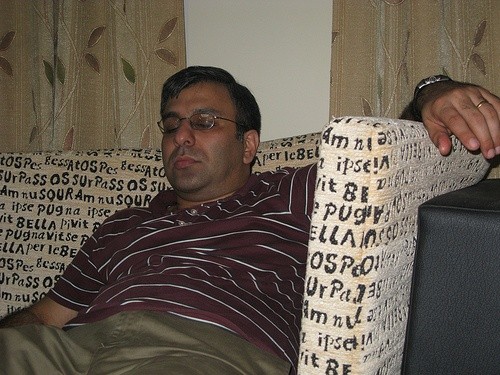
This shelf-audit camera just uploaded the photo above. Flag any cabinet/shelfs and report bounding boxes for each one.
[402,178,500,375]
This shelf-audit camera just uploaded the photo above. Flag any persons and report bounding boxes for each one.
[0,66,500,375]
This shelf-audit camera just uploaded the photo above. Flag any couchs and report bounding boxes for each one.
[0,116,495,375]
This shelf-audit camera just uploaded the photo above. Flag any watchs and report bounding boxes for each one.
[412,74,453,122]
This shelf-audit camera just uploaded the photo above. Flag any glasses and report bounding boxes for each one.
[157,113,237,134]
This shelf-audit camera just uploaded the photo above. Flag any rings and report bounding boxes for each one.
[476,99,489,109]
